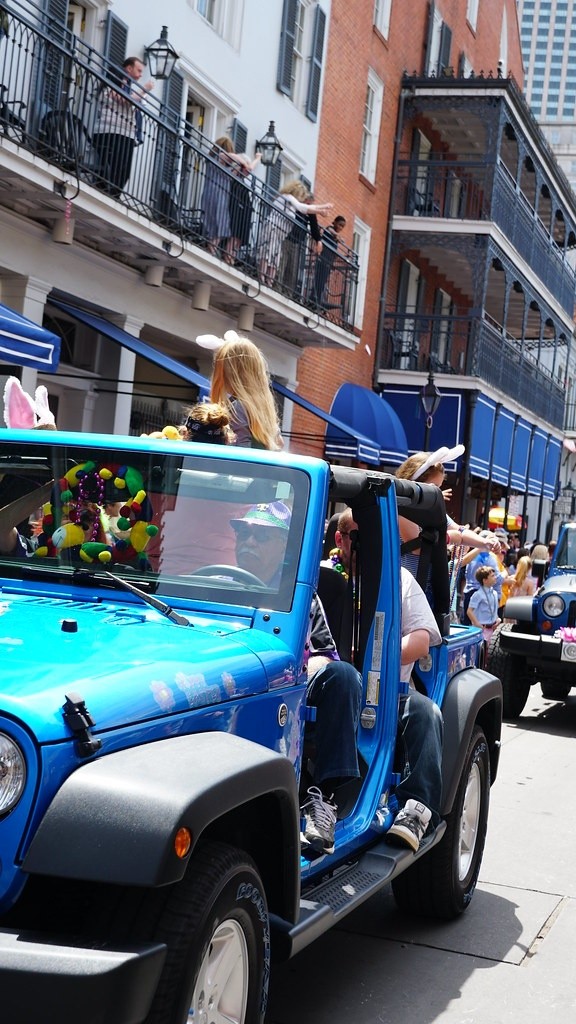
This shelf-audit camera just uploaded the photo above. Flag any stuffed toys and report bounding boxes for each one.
[33,460,159,571]
[52,522,93,563]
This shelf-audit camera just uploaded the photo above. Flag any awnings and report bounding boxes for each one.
[47,296,211,403]
[268,378,380,466]
[325,383,409,466]
[0,303,61,374]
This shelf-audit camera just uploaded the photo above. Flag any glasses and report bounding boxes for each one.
[235,528,270,542]
[340,530,358,539]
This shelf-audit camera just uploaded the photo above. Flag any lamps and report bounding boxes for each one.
[255,120,283,167]
[562,482,576,497]
[50,217,76,246]
[142,25,181,81]
[144,265,165,287]
[191,283,212,312]
[236,305,256,334]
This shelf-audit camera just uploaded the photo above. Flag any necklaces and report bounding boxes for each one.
[76,472,105,542]
[330,548,361,615]
[447,526,464,612]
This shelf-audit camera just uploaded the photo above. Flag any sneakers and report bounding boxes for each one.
[300,786,338,854]
[387,799,432,852]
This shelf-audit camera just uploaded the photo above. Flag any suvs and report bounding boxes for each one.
[485,523,576,719]
[0,426,507,1024]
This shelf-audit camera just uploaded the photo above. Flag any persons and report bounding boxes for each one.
[320,507,444,852]
[92,57,155,199]
[0,329,284,580]
[207,501,362,862]
[200,136,262,266]
[258,180,347,316]
[462,526,558,665]
[394,444,502,613]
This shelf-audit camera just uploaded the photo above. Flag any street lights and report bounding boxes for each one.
[419,370,443,451]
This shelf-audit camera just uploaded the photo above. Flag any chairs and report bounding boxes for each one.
[384,327,419,372]
[427,353,456,374]
[154,181,206,236]
[316,566,353,664]
[38,110,97,175]
[406,181,440,217]
[0,83,28,144]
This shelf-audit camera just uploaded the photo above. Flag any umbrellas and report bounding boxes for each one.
[482,503,527,530]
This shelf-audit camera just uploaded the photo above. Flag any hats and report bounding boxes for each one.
[531,545,549,561]
[496,531,510,549]
[494,528,509,535]
[230,501,292,533]
